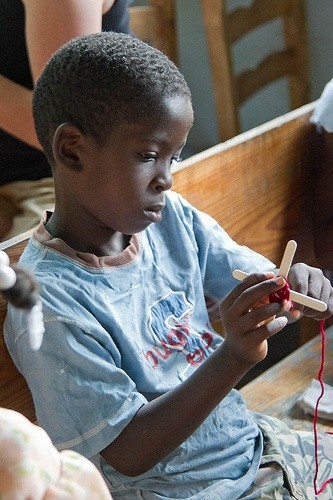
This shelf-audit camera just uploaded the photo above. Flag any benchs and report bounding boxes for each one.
[0,98,333,437]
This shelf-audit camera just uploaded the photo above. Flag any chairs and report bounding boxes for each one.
[129,0,180,74]
[201,0,310,144]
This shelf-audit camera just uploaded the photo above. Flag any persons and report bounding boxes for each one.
[0,0,132,243]
[308,73,333,273]
[3,29,333,500]
